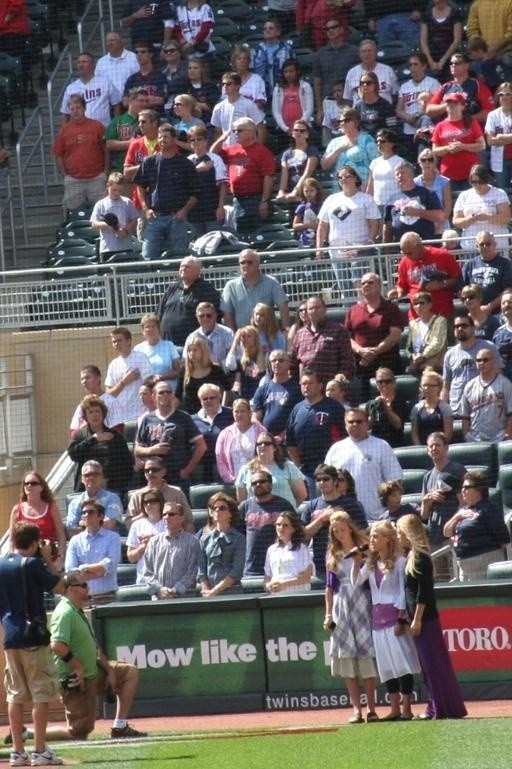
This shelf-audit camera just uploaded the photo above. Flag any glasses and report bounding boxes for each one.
[24,481,40,486]
[81,509,97,515]
[336,174,354,180]
[199,313,215,319]
[361,81,372,85]
[341,118,352,122]
[239,260,256,265]
[294,128,306,133]
[272,359,287,363]
[144,468,163,473]
[164,48,177,54]
[377,379,392,385]
[377,140,390,146]
[257,441,273,446]
[316,477,333,481]
[498,91,509,97]
[203,397,217,400]
[158,390,171,394]
[252,479,271,486]
[450,62,463,66]
[476,358,488,363]
[455,324,470,328]
[478,242,489,247]
[462,296,475,301]
[163,513,181,517]
[232,129,248,133]
[71,583,88,589]
[420,158,435,163]
[213,506,228,511]
[142,498,159,505]
[362,281,376,285]
[222,83,234,86]
[325,25,340,31]
[414,302,427,306]
[298,309,306,312]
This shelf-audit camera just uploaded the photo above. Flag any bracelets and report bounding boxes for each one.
[325,613,334,618]
[396,617,409,625]
[59,650,73,663]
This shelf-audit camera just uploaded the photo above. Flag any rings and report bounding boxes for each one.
[323,624,326,628]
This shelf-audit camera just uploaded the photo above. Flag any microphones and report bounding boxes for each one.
[344,544,368,559]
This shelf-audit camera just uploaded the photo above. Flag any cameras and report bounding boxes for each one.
[38,541,59,558]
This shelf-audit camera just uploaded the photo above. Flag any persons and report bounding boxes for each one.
[390,512,470,719]
[2,573,149,744]
[343,517,424,720]
[46,0,511,606]
[0,523,66,767]
[6,468,66,573]
[1,1,32,60]
[321,511,383,723]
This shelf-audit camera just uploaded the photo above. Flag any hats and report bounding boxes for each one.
[445,93,465,105]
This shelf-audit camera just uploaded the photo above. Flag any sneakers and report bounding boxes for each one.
[349,714,364,723]
[366,712,379,721]
[380,713,399,721]
[4,725,26,743]
[28,752,63,766]
[111,724,148,739]
[10,751,30,766]
[401,714,414,720]
[418,713,432,719]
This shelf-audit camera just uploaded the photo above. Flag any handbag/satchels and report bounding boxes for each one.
[23,619,52,646]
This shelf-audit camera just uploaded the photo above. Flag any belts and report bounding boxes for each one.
[326,97,334,100]
